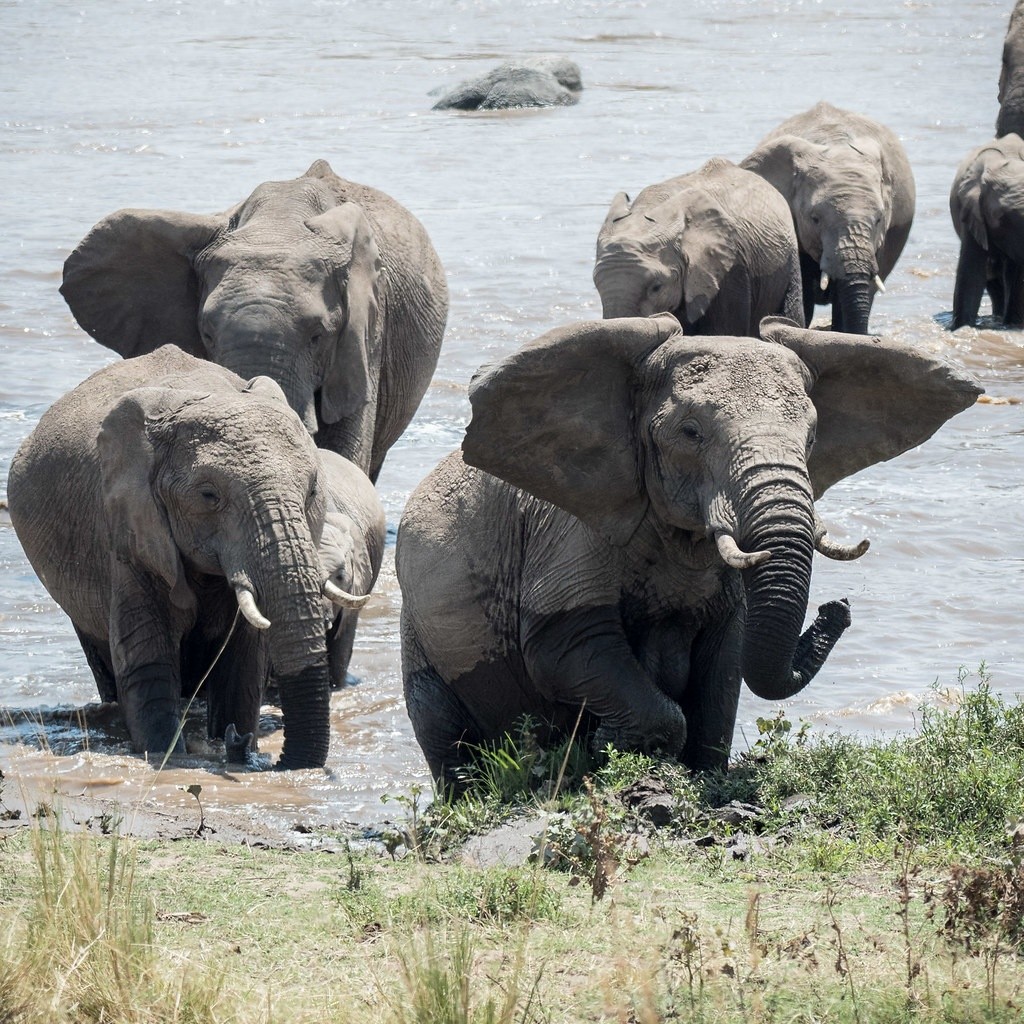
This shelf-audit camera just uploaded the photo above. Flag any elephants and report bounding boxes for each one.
[945,0,1024,331]
[394,98,986,817]
[5,157,450,774]
